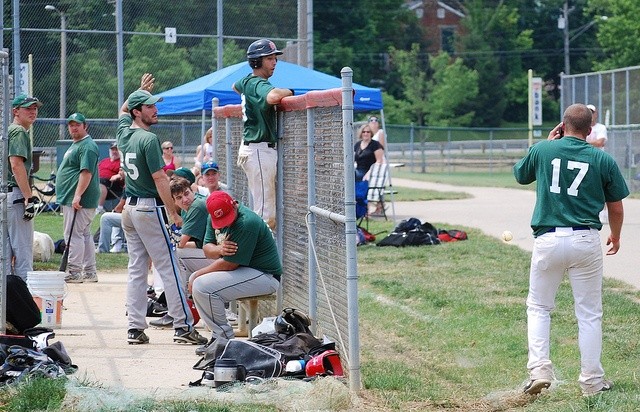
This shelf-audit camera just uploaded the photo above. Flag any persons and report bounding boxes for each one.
[165,167,206,220]
[96,167,125,253]
[96,143,121,214]
[200,161,229,197]
[115,72,208,344]
[187,191,283,356]
[368,116,389,219]
[587,103,610,227]
[161,141,181,173]
[513,102,630,397]
[231,39,295,230]
[6,93,43,282]
[353,124,385,182]
[193,126,212,167]
[54,113,101,283]
[149,177,217,327]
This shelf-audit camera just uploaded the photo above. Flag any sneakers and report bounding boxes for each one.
[65,273,83,283]
[128,329,149,344]
[523,380,551,395]
[82,272,98,282]
[174,328,208,345]
[598,380,615,391]
[109,243,125,253]
[195,336,216,357]
[149,314,174,330]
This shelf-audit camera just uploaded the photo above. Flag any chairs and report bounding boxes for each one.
[357,161,389,228]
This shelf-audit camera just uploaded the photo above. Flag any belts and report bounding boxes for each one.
[548,225,590,231]
[244,141,275,147]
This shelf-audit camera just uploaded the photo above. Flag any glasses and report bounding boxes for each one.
[16,97,39,108]
[163,147,173,150]
[370,119,378,121]
[362,131,370,133]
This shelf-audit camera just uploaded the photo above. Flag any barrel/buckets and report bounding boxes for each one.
[26,271,67,328]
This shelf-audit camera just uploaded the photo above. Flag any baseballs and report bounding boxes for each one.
[502,230,513,241]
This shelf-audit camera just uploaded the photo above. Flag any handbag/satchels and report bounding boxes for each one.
[376,232,408,246]
[396,218,439,245]
[221,332,322,379]
[437,230,467,242]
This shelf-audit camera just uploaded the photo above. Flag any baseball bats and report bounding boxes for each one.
[59,207,78,272]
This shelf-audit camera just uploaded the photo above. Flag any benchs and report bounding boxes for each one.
[227,297,276,338]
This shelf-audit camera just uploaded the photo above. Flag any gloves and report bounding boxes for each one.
[169,224,182,251]
[23,198,34,221]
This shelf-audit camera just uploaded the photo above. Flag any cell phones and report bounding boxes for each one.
[555,124,563,140]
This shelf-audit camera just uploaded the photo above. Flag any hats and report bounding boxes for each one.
[12,95,42,108]
[167,167,195,183]
[66,112,85,123]
[128,90,164,110]
[586,104,595,112]
[110,142,117,148]
[206,190,235,229]
[201,162,219,175]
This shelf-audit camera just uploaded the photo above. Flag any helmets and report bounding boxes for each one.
[247,38,284,68]
[305,350,343,377]
